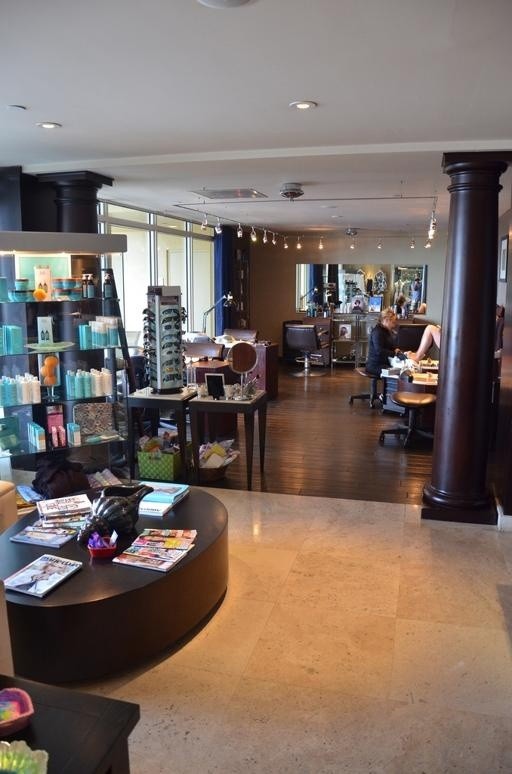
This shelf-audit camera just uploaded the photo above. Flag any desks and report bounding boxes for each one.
[0,672,140,774]
[245,340,279,401]
[0,479,227,674]
[127,386,267,483]
[193,361,230,385]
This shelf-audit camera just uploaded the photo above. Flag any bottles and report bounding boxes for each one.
[52,425,66,447]
[65,367,113,399]
[26,420,46,451]
[67,422,82,446]
[78,315,119,351]
[0,277,8,303]
[0,372,42,407]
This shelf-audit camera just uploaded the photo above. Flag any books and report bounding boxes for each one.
[111,529,198,573]
[87,469,122,490]
[134,481,190,517]
[16,486,45,516]
[10,493,93,549]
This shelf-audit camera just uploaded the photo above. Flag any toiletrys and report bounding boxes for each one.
[27,422,81,453]
[66,368,112,399]
[78,316,117,351]
[8,277,31,302]
[102,272,112,297]
[51,277,82,300]
[0,372,41,405]
[80,271,95,299]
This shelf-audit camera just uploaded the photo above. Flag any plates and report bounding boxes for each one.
[24,342,75,352]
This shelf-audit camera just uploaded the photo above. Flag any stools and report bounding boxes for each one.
[349,368,383,408]
[0,479,17,531]
[379,392,437,447]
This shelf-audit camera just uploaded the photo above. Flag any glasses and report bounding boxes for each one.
[163,367,179,372]
[163,374,182,382]
[145,367,156,374]
[143,326,154,333]
[161,316,178,323]
[145,359,157,365]
[163,325,179,330]
[143,342,150,346]
[179,312,185,316]
[161,334,179,341]
[163,350,178,355]
[183,362,186,368]
[177,330,186,335]
[162,309,179,315]
[179,317,185,324]
[143,317,155,324]
[142,349,156,358]
[179,307,185,312]
[162,342,179,349]
[183,347,187,353]
[143,334,155,340]
[142,309,155,317]
[148,375,157,382]
[162,358,179,366]
[182,356,185,360]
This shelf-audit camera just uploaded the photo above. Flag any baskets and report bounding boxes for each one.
[190,465,227,481]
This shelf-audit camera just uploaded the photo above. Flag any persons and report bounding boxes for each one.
[395,278,426,318]
[3,553,84,599]
[14,558,71,592]
[352,310,441,402]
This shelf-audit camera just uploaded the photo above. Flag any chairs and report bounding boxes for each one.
[392,318,434,350]
[224,328,258,340]
[182,344,225,359]
[283,320,328,378]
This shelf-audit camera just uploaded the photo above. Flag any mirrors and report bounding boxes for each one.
[226,342,258,400]
[295,263,427,315]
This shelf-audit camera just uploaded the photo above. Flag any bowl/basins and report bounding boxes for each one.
[87,536,118,559]
[0,687,36,737]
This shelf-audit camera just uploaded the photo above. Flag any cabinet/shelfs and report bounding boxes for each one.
[331,313,380,376]
[302,317,330,367]
[1,230,145,511]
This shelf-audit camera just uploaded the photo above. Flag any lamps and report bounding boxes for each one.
[300,287,318,308]
[280,183,304,202]
[200,210,438,250]
[203,292,237,333]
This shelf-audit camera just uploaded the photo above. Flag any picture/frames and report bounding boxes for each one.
[339,323,351,339]
[499,233,509,282]
